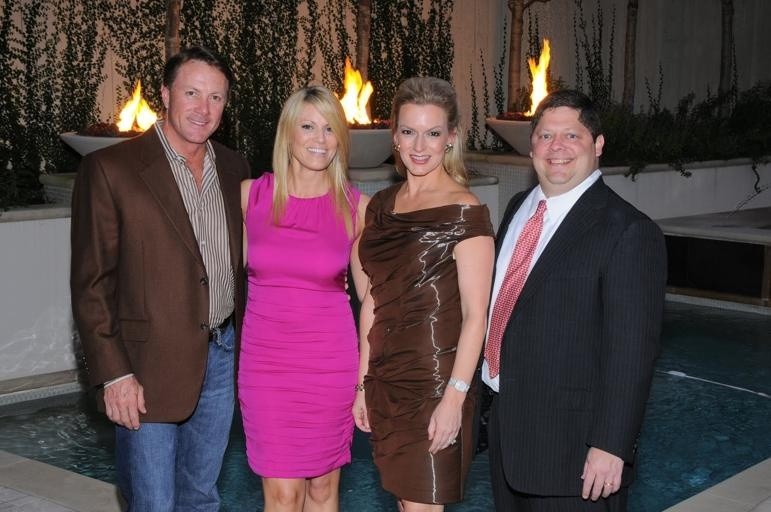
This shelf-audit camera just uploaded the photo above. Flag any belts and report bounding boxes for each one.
[210,316,232,342]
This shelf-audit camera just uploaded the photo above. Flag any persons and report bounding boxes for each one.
[348,73,497,512]
[68,46,252,512]
[237,84,374,512]
[474,89,670,510]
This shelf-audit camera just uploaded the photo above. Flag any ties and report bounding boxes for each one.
[483,200,547,379]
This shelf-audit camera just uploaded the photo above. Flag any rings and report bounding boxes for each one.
[448,437,458,445]
[604,480,616,487]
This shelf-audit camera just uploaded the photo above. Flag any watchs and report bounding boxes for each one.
[446,377,471,395]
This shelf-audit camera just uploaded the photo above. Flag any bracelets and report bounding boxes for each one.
[354,381,367,393]
